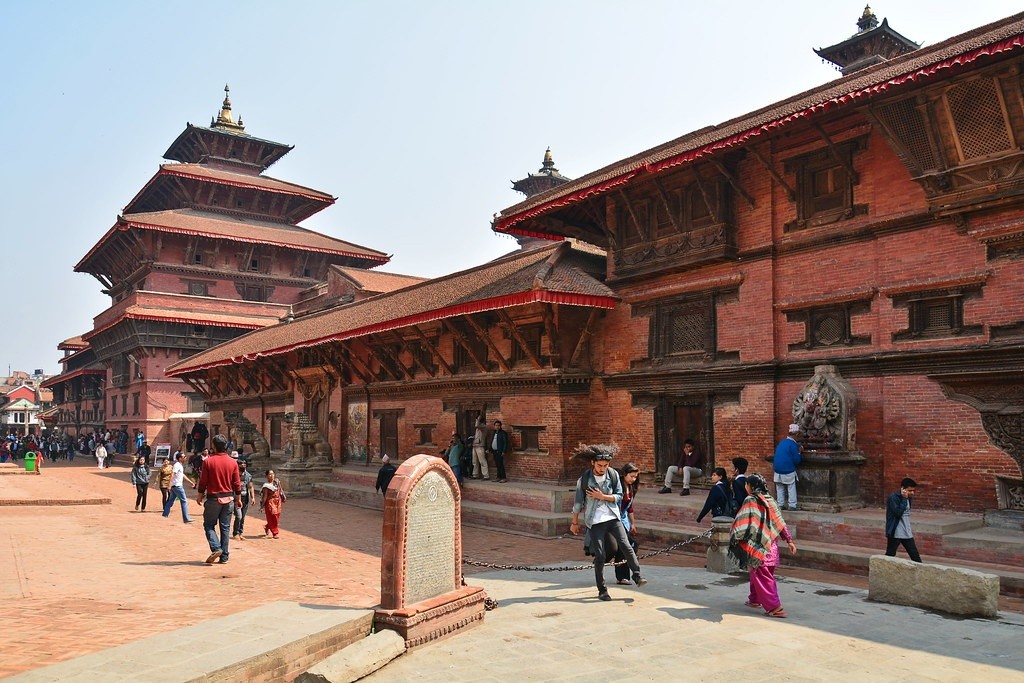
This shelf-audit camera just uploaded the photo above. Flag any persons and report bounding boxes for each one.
[696,468,734,524]
[485,421,508,483]
[467,415,490,480]
[439,434,477,488]
[195,433,244,564]
[727,472,796,617]
[658,439,703,495]
[375,452,396,498]
[884,477,922,563]
[729,458,750,573]
[568,441,648,601]
[583,462,639,585]
[774,424,804,511]
[0,420,287,539]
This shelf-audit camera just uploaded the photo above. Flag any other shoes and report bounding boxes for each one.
[218,559,228,563]
[234,534,246,540]
[263,525,269,535]
[765,609,787,618]
[273,534,279,539]
[658,487,672,494]
[448,471,512,488]
[632,574,647,586]
[744,601,762,607]
[206,550,222,562]
[680,489,690,495]
[599,592,611,601]
[135,506,146,512]
[184,518,195,523]
[779,506,801,510]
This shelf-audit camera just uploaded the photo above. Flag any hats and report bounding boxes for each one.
[788,424,799,432]
[230,451,239,458]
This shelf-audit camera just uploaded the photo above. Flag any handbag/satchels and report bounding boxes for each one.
[621,511,631,532]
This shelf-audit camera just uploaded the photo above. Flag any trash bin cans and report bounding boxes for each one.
[24,452,37,471]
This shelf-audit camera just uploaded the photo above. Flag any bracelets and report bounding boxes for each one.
[572,522,579,526]
